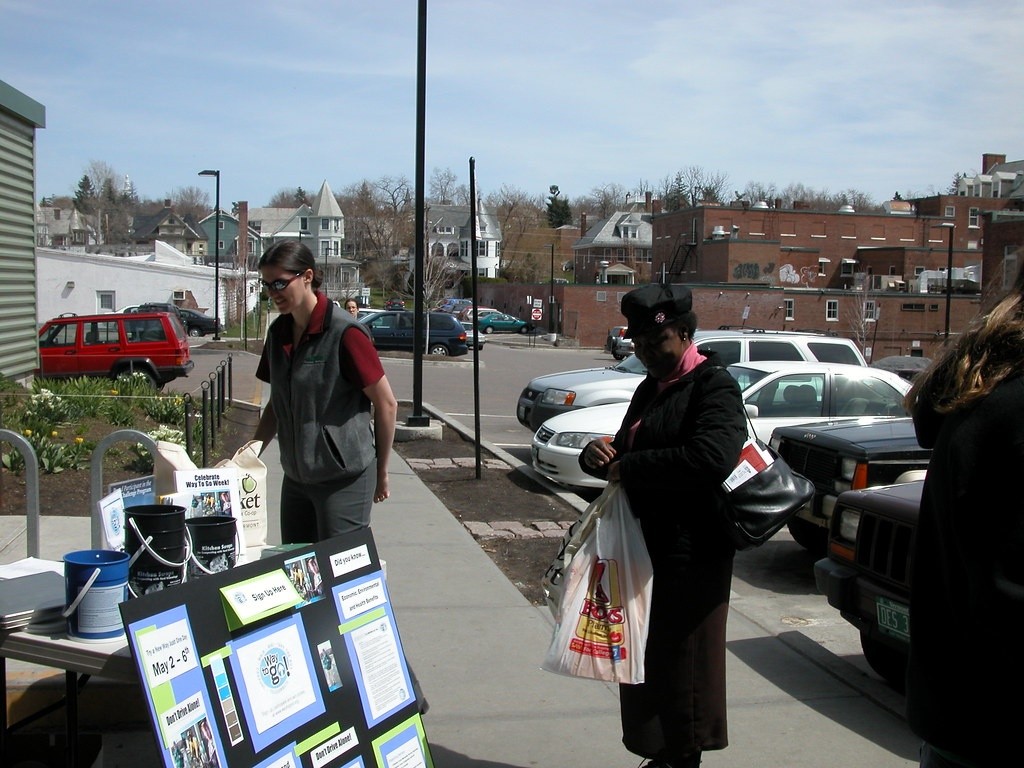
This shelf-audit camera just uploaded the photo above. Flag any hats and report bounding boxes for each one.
[621,283,692,340]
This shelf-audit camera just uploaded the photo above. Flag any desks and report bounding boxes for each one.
[0,624,163,768]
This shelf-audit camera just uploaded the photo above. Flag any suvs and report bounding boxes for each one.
[606,325,635,360]
[38,311,195,395]
[515,328,874,437]
[358,310,469,357]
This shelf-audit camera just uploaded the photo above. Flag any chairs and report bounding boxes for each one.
[775,385,798,417]
[791,385,820,416]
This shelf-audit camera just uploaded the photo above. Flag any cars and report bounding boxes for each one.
[384,298,406,310]
[868,355,932,383]
[101,302,224,343]
[356,307,384,325]
[765,416,934,558]
[430,297,535,351]
[529,359,920,495]
[810,468,931,688]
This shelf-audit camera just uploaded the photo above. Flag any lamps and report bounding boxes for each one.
[67,281,75,288]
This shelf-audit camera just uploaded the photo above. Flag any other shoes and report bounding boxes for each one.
[638,755,700,768]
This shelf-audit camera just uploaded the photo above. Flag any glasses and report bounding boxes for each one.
[261,269,307,293]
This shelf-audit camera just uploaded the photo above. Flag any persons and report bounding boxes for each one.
[251,239,397,544]
[193,493,231,517]
[344,298,359,318]
[902,257,1024,768]
[171,720,218,768]
[578,281,748,768]
[286,556,323,599]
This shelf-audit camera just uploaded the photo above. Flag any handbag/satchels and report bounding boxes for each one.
[688,365,815,547]
[541,480,655,683]
[208,439,269,546]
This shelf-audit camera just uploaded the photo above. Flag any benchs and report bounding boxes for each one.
[837,398,906,417]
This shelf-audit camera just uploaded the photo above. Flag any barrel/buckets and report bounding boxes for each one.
[123,504,194,601]
[63,548,131,644]
[183,515,240,584]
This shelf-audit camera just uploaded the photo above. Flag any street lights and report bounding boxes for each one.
[325,247,333,293]
[197,169,220,340]
[299,230,311,242]
[939,222,954,346]
